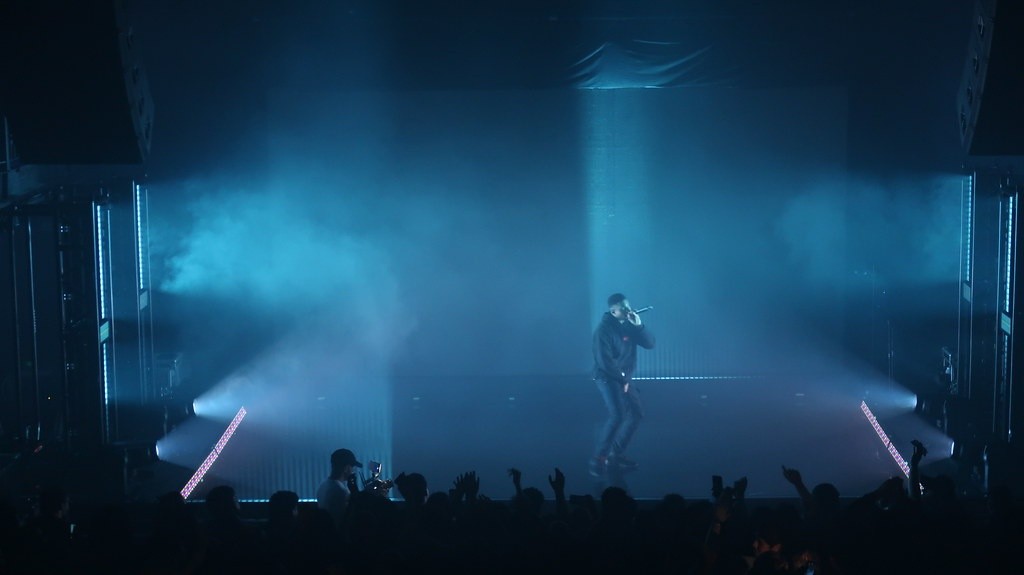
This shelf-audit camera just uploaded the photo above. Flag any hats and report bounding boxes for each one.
[333,447,363,468]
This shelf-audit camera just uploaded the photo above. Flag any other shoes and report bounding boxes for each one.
[587,457,603,476]
[605,455,639,468]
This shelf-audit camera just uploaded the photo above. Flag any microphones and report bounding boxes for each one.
[628,305,654,317]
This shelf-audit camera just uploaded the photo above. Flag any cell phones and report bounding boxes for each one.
[712,476,723,499]
[911,439,928,456]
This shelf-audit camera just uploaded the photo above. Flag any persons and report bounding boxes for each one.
[314,448,364,525]
[584,293,656,477]
[0,443,1024,575]
